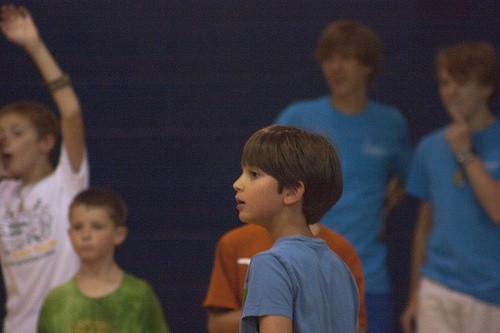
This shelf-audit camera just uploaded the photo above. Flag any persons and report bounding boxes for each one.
[270,20,417,333]
[37,188,165,332]
[205,222,366,332]
[0,3,89,333]
[402,40,500,332]
[233,125,360,333]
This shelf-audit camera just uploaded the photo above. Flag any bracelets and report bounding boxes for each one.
[455,146,477,164]
[46,74,72,93]
